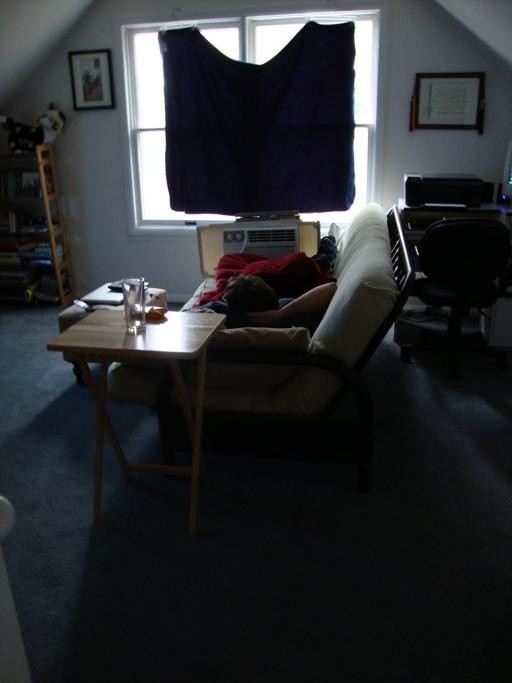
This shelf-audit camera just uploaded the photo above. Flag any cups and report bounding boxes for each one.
[122,279,147,332]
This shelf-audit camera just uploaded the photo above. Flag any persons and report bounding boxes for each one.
[177,272,338,336]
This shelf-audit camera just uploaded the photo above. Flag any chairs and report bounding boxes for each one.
[401,216,511,360]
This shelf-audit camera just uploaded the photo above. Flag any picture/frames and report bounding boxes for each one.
[69,49,116,112]
[410,70,486,135]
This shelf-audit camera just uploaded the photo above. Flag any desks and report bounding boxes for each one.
[46,307,227,541]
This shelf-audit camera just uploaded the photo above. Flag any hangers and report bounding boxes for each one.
[306,1,358,25]
[156,7,200,38]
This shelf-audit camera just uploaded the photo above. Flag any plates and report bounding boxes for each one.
[130,304,166,320]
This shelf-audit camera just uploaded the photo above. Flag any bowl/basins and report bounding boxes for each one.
[129,288,168,309]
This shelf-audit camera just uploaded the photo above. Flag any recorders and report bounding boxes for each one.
[222,221,298,257]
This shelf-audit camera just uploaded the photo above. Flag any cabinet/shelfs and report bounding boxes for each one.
[1,141,77,311]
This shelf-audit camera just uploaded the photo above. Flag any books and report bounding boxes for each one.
[1,167,72,304]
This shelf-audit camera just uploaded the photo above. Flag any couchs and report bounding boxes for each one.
[157,203,408,469]
